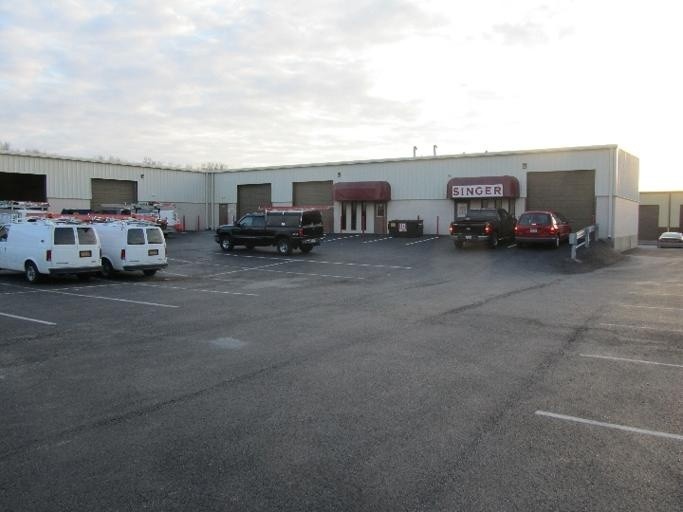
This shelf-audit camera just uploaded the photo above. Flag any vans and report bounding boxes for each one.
[0,199,184,284]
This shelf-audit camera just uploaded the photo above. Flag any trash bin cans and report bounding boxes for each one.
[388,220,423,237]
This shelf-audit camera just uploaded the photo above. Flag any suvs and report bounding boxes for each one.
[214,208,327,257]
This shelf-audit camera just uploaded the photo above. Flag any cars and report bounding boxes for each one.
[658,231,683,249]
[514,211,571,249]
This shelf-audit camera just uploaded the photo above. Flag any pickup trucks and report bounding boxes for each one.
[447,208,517,249]
